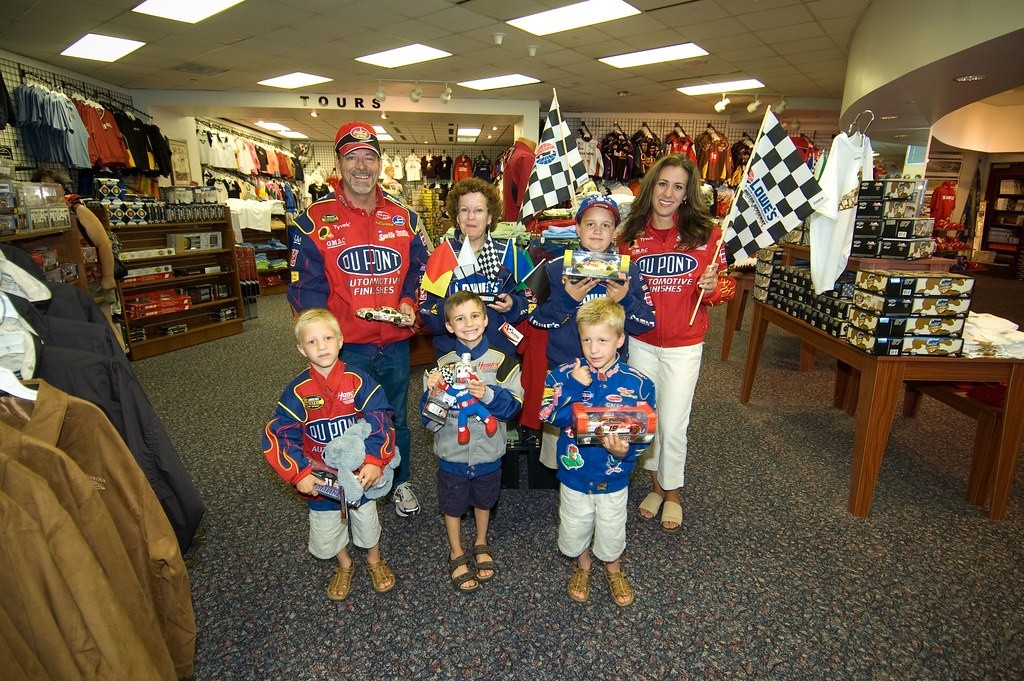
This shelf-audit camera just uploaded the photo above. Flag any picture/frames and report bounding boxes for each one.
[48,206,72,230]
[26,205,51,230]
[165,135,192,186]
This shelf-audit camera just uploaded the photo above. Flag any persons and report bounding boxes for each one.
[308,177,330,204]
[32,169,127,353]
[419,291,525,594]
[423,179,536,374]
[616,154,737,533]
[538,297,657,607]
[383,167,398,183]
[261,308,396,601]
[503,138,536,223]
[290,122,429,518]
[436,363,498,444]
[528,193,657,470]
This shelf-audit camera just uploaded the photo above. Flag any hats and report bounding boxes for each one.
[335,121,381,158]
[576,194,621,224]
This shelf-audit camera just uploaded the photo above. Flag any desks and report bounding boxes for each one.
[778,242,958,408]
[740,297,1024,522]
[720,272,820,372]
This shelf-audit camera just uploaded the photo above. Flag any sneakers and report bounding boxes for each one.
[386,481,421,517]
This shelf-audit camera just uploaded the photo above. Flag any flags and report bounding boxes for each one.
[722,110,827,265]
[422,234,535,298]
[516,98,589,226]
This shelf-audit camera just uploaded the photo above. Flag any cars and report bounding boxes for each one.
[588,411,646,435]
[571,257,618,277]
[355,306,410,326]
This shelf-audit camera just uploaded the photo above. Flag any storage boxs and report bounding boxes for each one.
[120,264,174,285]
[29,245,98,283]
[752,177,975,359]
[123,289,192,319]
[572,401,658,446]
[411,187,443,247]
[500,442,559,491]
[562,249,630,280]
[167,232,223,254]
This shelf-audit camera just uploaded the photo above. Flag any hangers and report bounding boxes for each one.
[71,84,103,111]
[705,122,722,144]
[24,71,70,101]
[642,124,659,146]
[614,123,625,140]
[578,122,592,142]
[741,135,755,148]
[0,250,38,401]
[381,148,487,163]
[674,125,689,142]
[105,94,155,124]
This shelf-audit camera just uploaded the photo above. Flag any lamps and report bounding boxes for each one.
[774,95,787,114]
[746,95,762,113]
[714,94,730,113]
[791,113,801,132]
[439,83,453,105]
[409,81,423,103]
[375,79,385,102]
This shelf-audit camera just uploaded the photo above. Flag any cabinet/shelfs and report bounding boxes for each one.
[75,202,247,360]
[241,212,292,296]
[0,214,104,303]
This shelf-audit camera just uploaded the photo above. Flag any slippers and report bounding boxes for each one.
[660,493,683,533]
[637,492,663,521]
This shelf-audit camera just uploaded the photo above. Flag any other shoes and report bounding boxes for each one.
[460,499,496,521]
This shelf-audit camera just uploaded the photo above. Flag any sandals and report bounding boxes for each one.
[471,542,495,582]
[366,556,395,593]
[566,563,592,602]
[604,567,633,607]
[448,550,479,593]
[327,558,355,601]
